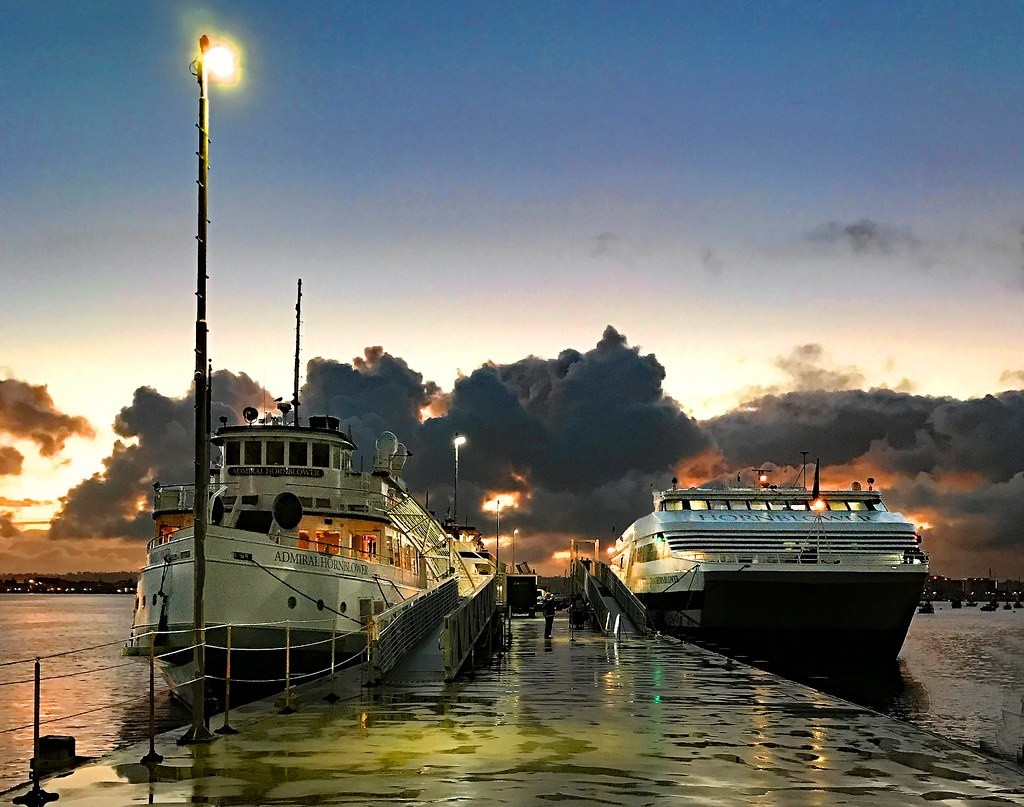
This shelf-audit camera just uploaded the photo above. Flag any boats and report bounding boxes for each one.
[611,452,930,663]
[1003,601,1012,610]
[951,600,962,609]
[917,602,934,614]
[120,275,436,713]
[917,601,923,607]
[965,600,978,607]
[985,599,999,607]
[1014,601,1022,608]
[980,606,996,611]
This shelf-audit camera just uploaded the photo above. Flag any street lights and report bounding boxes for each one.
[179,30,242,747]
[512,529,519,574]
[496,501,503,576]
[453,432,467,528]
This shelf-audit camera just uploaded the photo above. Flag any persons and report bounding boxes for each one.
[542,592,556,639]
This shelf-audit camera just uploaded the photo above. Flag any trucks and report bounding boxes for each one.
[506,574,537,618]
[536,589,546,610]
[552,592,563,610]
